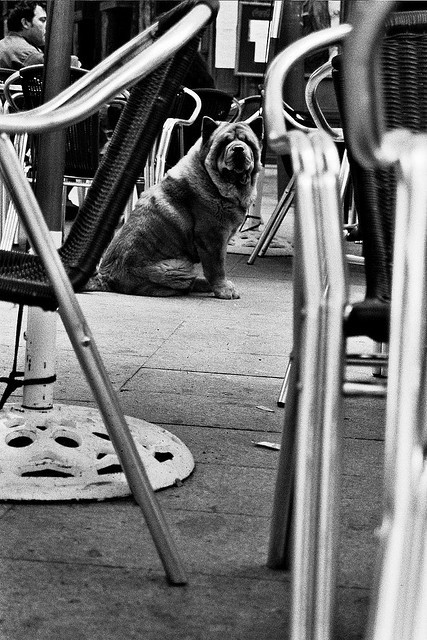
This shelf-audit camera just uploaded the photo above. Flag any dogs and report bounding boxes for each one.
[84,116,263,299]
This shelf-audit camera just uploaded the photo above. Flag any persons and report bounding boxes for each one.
[0,1,81,71]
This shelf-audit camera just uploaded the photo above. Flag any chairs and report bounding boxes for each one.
[331,1,426,638]
[19,64,138,250]
[0,67,42,101]
[149,86,202,188]
[197,86,242,131]
[232,95,272,165]
[262,21,392,640]
[246,82,313,265]
[0,1,224,584]
